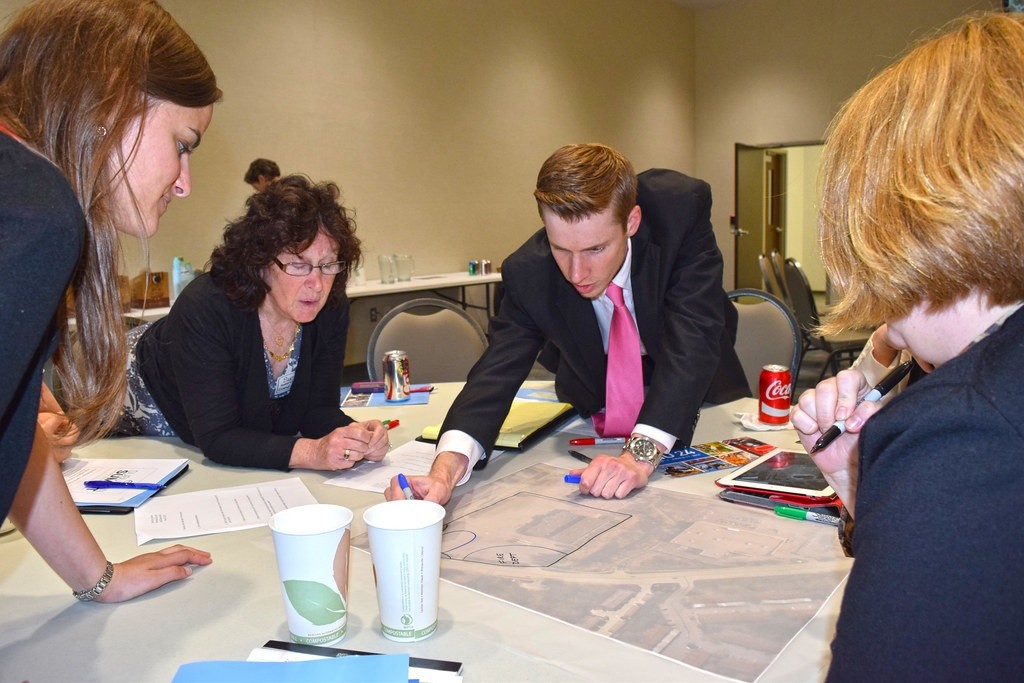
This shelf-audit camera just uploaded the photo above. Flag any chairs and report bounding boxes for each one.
[759,252,870,381]
[727,289,802,404]
[366,297,489,383]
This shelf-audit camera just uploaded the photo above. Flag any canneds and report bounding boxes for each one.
[468,259,491,276]
[381,350,410,402]
[758,364,792,426]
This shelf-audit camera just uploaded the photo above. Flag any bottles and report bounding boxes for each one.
[169,270,195,309]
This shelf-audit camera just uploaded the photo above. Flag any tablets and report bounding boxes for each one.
[715,447,838,502]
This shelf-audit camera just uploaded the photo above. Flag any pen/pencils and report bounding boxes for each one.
[383,419,400,431]
[85,480,166,490]
[808,360,915,455]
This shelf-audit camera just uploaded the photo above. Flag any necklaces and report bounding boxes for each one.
[264,322,300,361]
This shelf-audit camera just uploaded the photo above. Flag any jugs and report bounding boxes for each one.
[394,255,416,282]
[378,254,397,283]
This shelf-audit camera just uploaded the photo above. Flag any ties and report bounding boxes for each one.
[592,281,644,439]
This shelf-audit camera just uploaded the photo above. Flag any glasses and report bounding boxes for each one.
[271,256,346,276]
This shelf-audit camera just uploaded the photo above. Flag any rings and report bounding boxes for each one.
[344,450,350,460]
[389,442,392,448]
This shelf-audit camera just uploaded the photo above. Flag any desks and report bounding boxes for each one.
[346,273,503,338]
[0,382,855,683]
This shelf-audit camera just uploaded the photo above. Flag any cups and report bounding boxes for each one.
[268,503,355,648]
[362,499,447,644]
[355,266,365,285]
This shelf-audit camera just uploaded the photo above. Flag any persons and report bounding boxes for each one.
[0,0,224,604]
[384,144,753,505]
[87,159,389,473]
[37,381,80,464]
[789,13,1024,683]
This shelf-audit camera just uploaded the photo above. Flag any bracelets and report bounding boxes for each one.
[73,561,114,602]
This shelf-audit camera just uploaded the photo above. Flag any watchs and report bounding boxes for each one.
[623,436,661,470]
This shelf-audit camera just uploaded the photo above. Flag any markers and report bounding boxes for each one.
[569,437,629,444]
[774,508,842,527]
[398,472,415,499]
